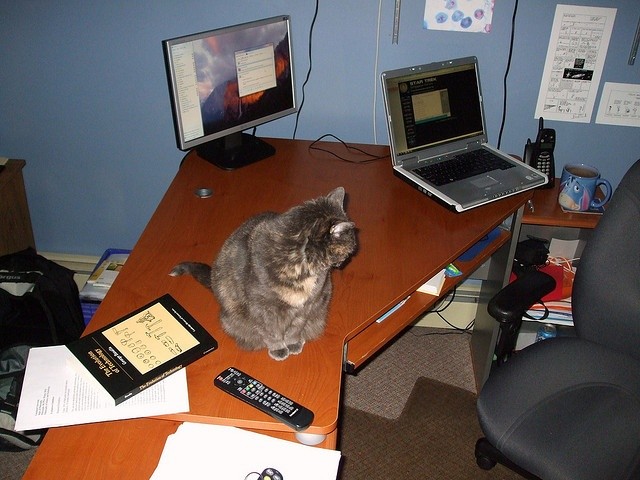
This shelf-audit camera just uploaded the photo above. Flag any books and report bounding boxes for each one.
[65,294,218,408]
[522,265,575,327]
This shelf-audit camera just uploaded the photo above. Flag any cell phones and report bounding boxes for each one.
[534,116,556,178]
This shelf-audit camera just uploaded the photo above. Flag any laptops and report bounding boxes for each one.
[381,55,549,213]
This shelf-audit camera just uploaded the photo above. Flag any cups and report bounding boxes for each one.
[558,163,611,211]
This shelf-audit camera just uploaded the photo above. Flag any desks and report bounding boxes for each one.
[19,137,535,480]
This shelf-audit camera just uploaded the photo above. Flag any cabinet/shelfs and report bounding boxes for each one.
[1,158,38,257]
[496,177,611,354]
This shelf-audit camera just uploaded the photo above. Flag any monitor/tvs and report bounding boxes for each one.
[161,14,299,171]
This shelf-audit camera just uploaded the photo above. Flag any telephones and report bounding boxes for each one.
[525,117,556,190]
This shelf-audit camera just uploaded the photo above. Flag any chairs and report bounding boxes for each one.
[474,160,640,480]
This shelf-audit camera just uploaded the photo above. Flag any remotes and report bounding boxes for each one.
[213,368,314,432]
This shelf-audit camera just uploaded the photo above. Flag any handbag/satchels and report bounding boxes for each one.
[2,246,86,348]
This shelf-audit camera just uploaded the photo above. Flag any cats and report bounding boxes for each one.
[168,186,358,360]
